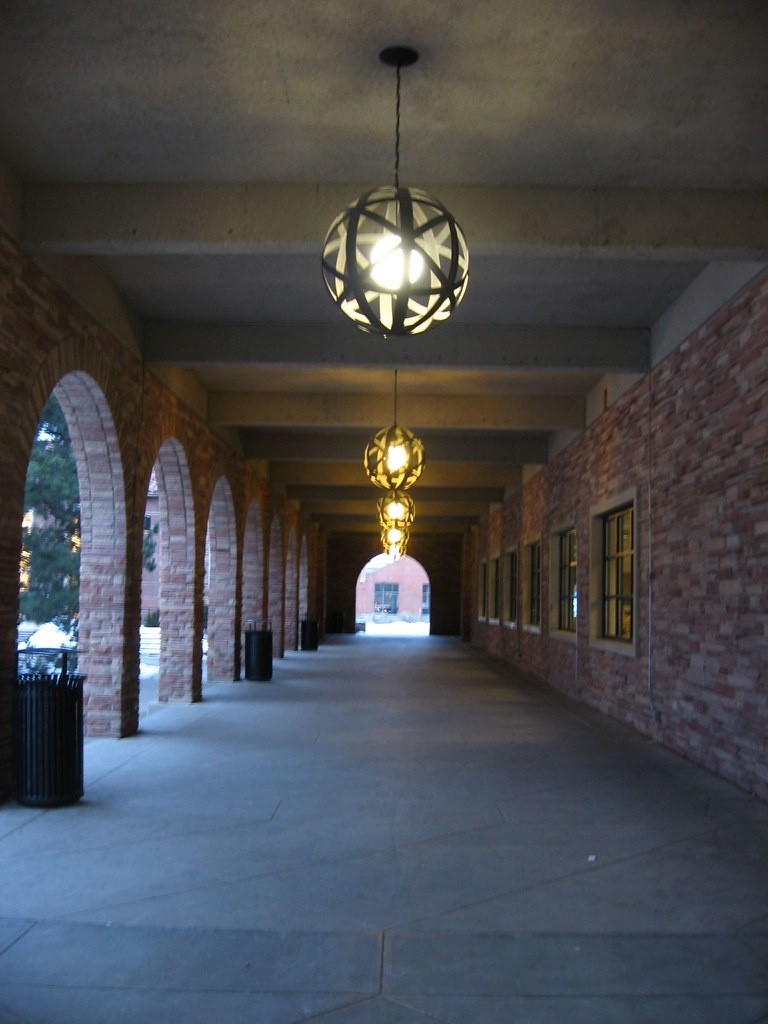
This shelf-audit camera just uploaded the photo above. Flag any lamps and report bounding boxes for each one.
[377,495,415,530]
[361,370,426,492]
[321,45,473,335]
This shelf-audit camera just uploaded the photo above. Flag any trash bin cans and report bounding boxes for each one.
[299,612,321,650]
[242,619,276,681]
[8,648,89,807]
